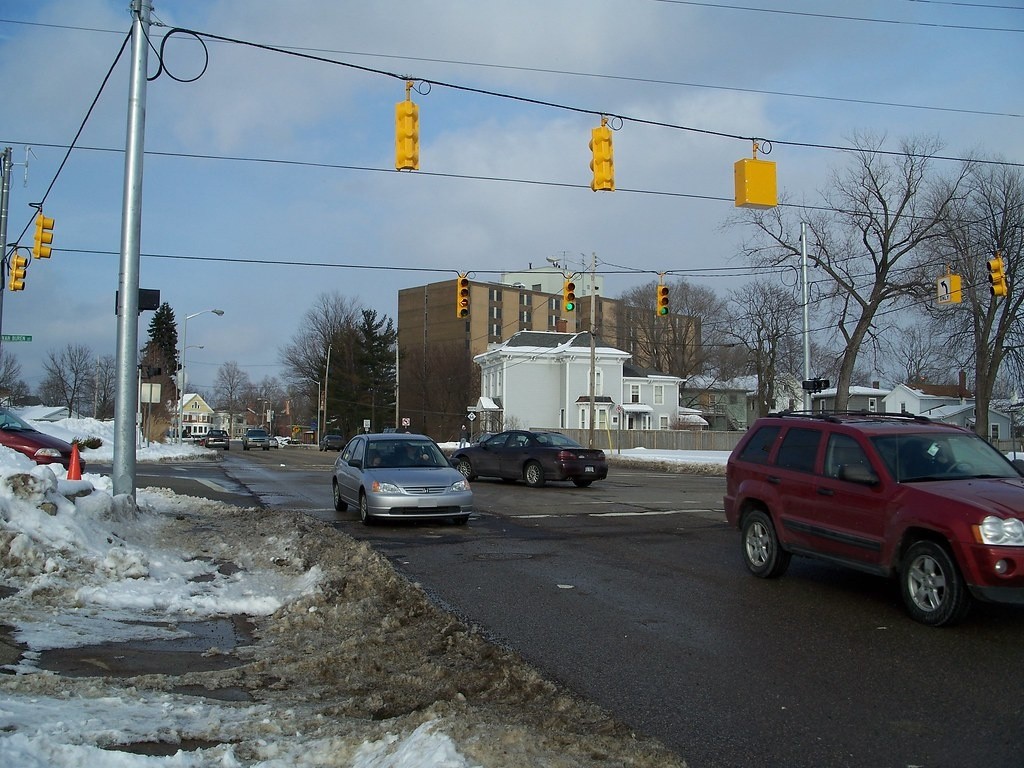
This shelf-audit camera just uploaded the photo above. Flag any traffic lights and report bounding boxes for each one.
[138,288,161,314]
[9,252,28,291]
[803,380,830,391]
[655,286,670,316]
[564,280,576,313]
[457,275,470,319]
[148,365,162,377]
[34,214,54,260]
[987,258,1007,297]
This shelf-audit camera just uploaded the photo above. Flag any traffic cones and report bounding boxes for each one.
[67,444,81,480]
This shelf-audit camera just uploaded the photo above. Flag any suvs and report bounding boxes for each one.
[723,409,1024,625]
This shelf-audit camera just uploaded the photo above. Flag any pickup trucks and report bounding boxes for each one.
[242,429,270,451]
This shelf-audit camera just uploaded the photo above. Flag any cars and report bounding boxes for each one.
[318,435,345,452]
[269,437,278,450]
[331,434,474,524]
[450,429,608,490]
[200,429,230,450]
[0,405,85,474]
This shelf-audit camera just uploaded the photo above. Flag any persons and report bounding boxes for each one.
[367,443,430,466]
[906,438,965,474]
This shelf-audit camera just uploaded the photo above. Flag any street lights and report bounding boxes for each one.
[258,378,320,444]
[176,308,226,446]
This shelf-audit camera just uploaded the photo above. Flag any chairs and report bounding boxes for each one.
[838,463,871,480]
